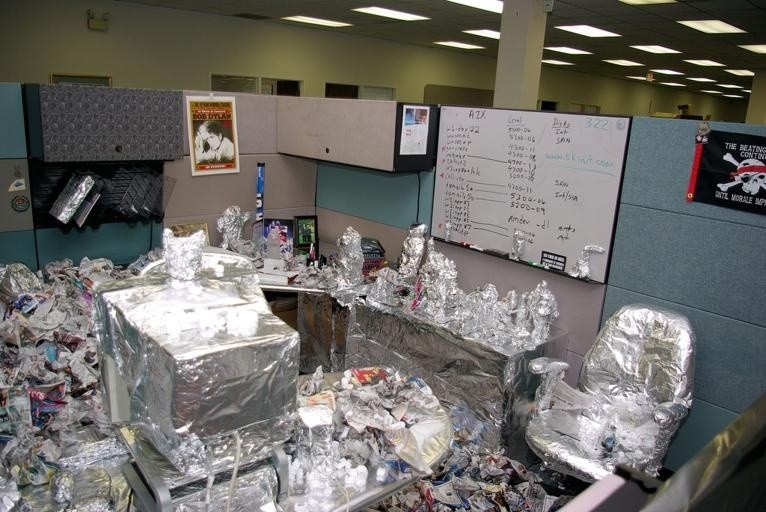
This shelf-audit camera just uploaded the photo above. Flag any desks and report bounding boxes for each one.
[116,363,454,512]
[249,252,356,372]
[359,276,569,467]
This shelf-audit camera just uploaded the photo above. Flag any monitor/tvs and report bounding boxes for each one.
[92,266,301,477]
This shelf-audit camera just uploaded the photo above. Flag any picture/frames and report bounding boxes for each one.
[261,217,293,252]
[292,214,320,250]
[186,95,242,176]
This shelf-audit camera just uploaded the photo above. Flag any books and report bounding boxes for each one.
[258,270,299,286]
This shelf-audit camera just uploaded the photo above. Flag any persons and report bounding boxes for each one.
[279,231,291,254]
[301,223,312,242]
[194,121,235,164]
[405,108,427,125]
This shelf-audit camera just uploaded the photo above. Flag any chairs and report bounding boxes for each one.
[528,303,696,483]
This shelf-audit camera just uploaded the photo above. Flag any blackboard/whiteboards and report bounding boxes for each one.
[429,104,633,283]
